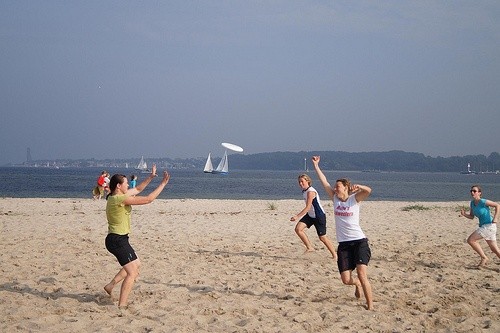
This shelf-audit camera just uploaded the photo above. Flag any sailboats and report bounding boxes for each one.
[134,158,152,175]
[211,150,230,176]
[203,150,213,173]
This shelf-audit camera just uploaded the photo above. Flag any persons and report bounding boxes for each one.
[104,164,170,307]
[94,171,137,200]
[290,174,338,260]
[311,155,373,310]
[461,185,500,267]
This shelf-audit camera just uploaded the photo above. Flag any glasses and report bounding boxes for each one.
[470,190,479,193]
